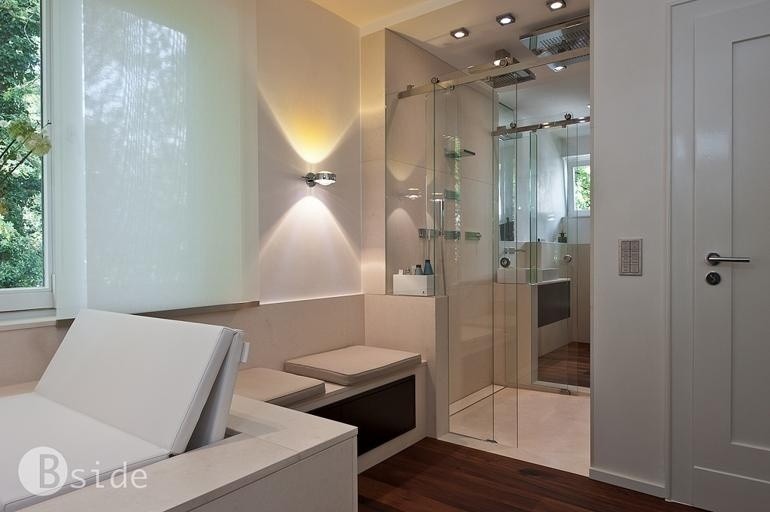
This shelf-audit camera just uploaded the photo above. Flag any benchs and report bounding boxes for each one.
[217,344,433,470]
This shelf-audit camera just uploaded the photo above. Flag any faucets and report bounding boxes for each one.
[504,246,527,254]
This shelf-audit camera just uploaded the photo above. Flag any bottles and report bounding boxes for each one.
[424,260,433,274]
[414,265,422,275]
[405,265,410,275]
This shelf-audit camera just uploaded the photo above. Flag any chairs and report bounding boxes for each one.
[0,308,358,511]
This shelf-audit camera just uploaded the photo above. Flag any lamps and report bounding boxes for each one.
[539,49,566,73]
[493,58,508,68]
[544,0,567,11]
[449,27,470,39]
[495,13,515,26]
[301,172,337,188]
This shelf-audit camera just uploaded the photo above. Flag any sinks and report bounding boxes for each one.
[496,266,561,284]
